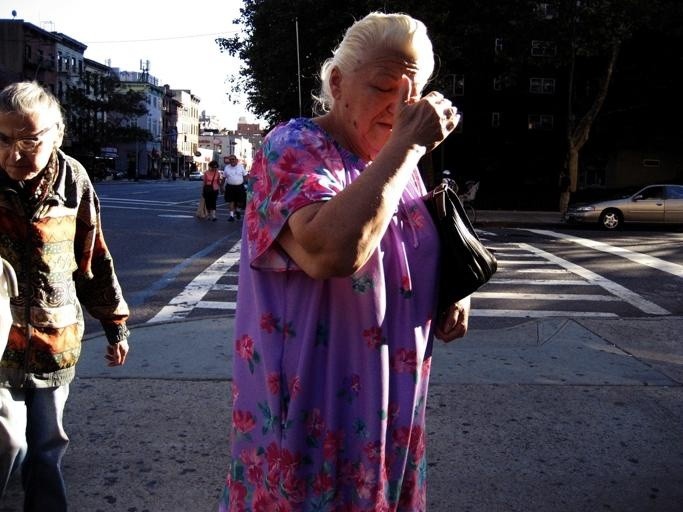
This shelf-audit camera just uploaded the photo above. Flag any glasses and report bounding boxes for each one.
[1,128,55,152]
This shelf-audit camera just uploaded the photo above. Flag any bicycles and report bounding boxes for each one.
[465,204,477,226]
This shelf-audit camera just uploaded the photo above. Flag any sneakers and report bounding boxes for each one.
[227,210,243,222]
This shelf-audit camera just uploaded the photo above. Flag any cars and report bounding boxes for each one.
[564,184,683,231]
[189,170,203,181]
[218,171,224,179]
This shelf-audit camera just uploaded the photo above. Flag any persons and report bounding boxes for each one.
[220,11,472,512]
[441,169,460,194]
[220,155,248,222]
[201,160,226,222]
[0,79,131,511]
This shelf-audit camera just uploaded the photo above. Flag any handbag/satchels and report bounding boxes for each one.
[421,178,498,313]
[202,184,215,200]
